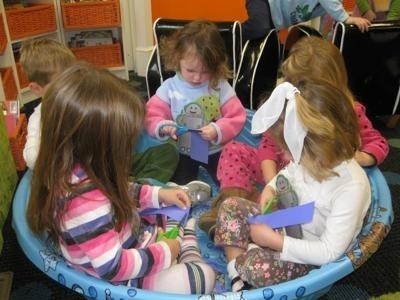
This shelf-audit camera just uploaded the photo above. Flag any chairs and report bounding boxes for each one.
[330,19,400,116]
[145,15,281,110]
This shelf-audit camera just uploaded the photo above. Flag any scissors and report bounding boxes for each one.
[252,195,279,217]
[156,212,187,243]
[171,125,202,137]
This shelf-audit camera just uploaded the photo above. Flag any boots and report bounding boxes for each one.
[198,186,250,232]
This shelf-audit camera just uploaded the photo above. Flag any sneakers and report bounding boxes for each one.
[165,180,213,208]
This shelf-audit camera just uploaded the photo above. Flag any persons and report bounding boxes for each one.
[353,0,400,21]
[144,17,247,185]
[237,0,373,41]
[213,76,372,293]
[25,59,216,295]
[19,38,180,185]
[197,36,390,240]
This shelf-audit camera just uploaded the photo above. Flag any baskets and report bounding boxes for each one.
[16,61,29,89]
[70,41,123,67]
[61,1,121,29]
[9,113,28,171]
[1,67,18,101]
[5,4,57,39]
